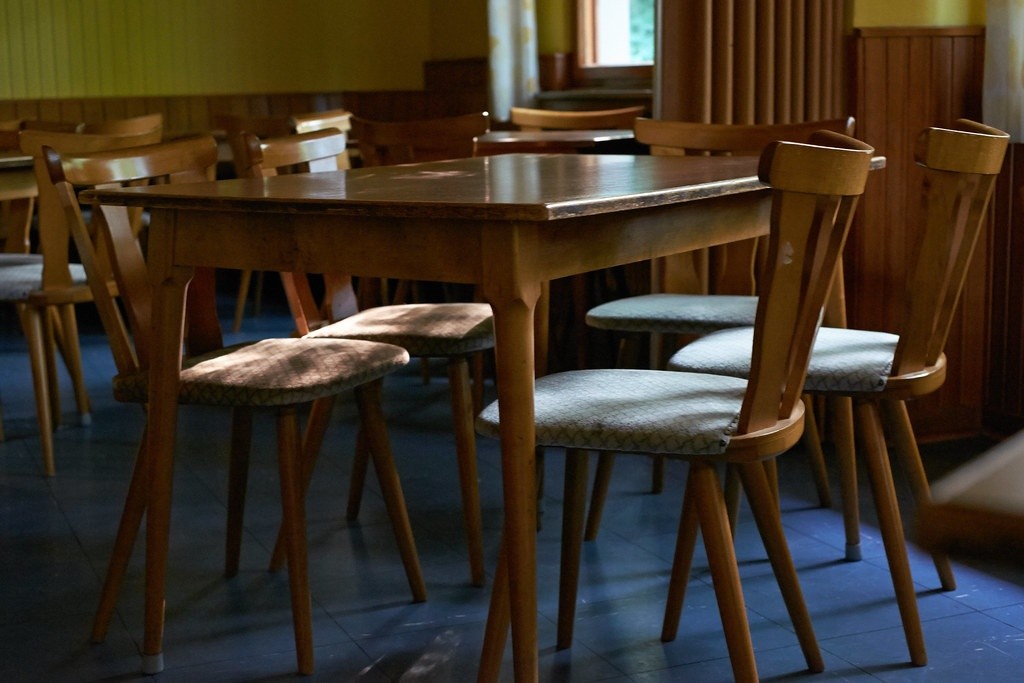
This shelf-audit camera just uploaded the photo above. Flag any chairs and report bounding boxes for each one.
[0,91,1011,683]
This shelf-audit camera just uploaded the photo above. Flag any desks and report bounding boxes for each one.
[78,148,891,683]
